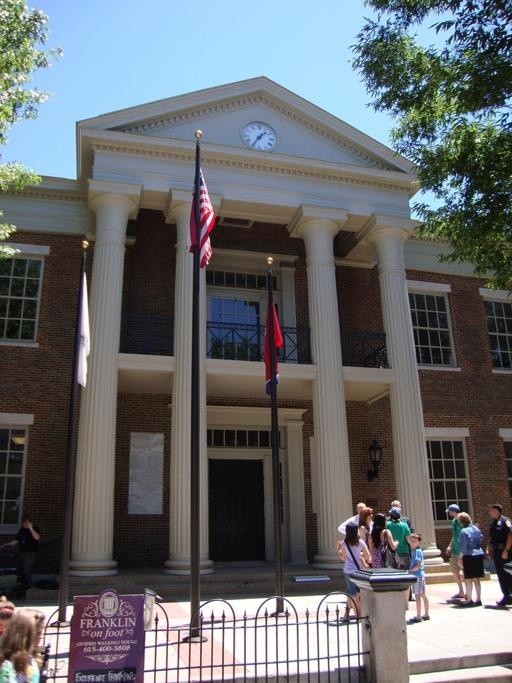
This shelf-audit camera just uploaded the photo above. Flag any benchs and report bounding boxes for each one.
[76,272,93,388]
[183,160,219,269]
[262,269,288,396]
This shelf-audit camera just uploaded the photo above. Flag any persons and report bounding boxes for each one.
[0,608,48,683]
[1,514,42,600]
[454,510,486,607]
[0,595,16,644]
[444,502,467,599]
[486,500,512,606]
[334,497,430,622]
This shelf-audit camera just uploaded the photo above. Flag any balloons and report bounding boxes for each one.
[239,119,282,153]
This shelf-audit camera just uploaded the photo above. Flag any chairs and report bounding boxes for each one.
[381,546,401,568]
[453,594,482,607]
[339,617,350,623]
[410,597,415,600]
[497,598,512,605]
[410,615,430,622]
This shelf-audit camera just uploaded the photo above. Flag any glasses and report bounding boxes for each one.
[366,439,383,482]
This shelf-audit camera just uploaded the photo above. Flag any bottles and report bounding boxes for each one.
[445,504,460,514]
[389,507,401,518]
